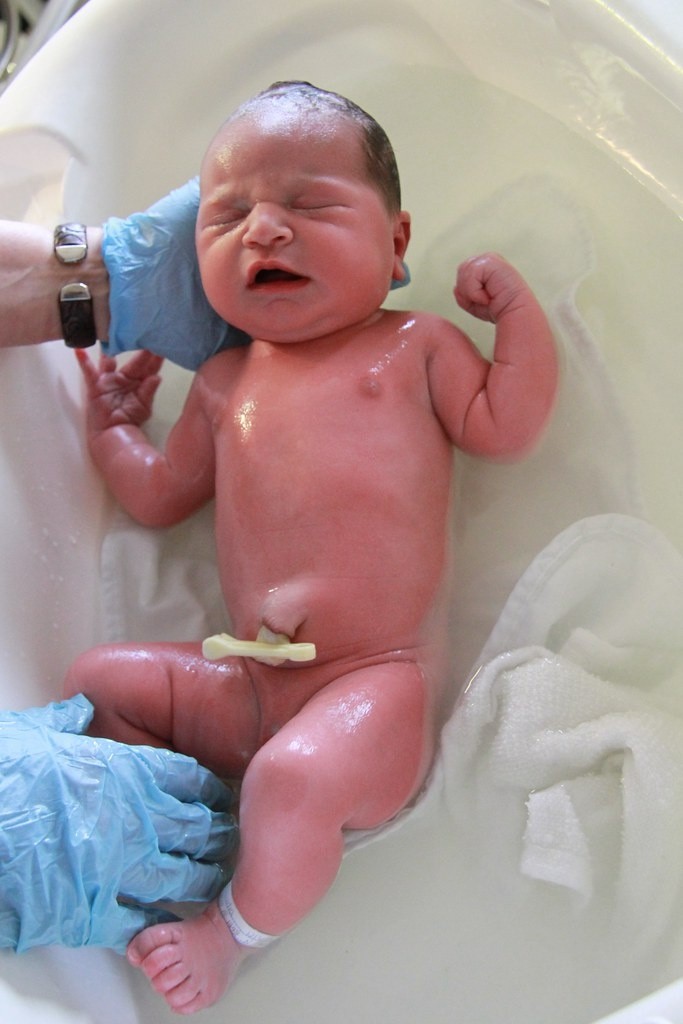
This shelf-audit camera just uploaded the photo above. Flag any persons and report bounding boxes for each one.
[3,693,244,962]
[0,171,410,369]
[66,81,562,1006]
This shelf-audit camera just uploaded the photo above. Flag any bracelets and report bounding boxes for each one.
[54,220,97,349]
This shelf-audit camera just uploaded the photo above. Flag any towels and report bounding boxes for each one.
[93,174,683,950]
